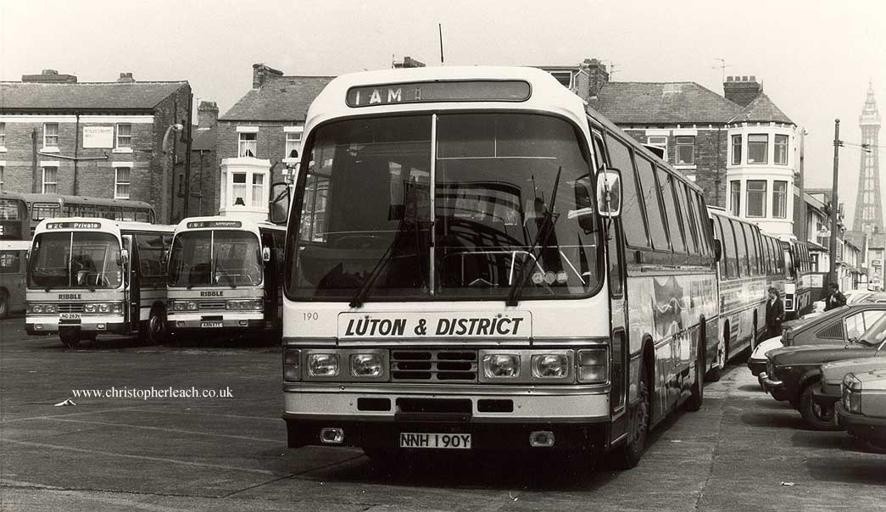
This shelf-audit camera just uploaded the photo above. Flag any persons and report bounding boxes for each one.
[825,282,846,311]
[765,287,784,338]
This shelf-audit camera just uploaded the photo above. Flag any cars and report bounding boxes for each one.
[748,290,886,449]
